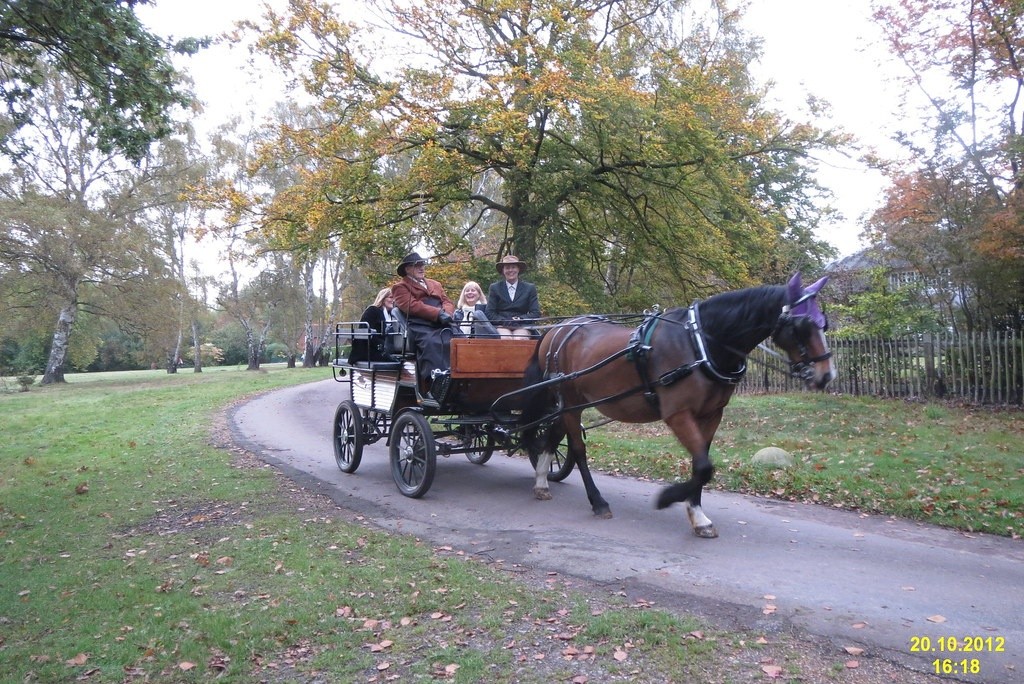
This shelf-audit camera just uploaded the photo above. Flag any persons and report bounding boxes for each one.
[452,280,489,338]
[392,252,466,399]
[349,287,398,366]
[485,255,542,340]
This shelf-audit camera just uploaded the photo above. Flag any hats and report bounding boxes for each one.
[496,255,527,274]
[397,252,425,278]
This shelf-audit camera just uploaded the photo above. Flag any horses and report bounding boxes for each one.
[519,270,837,539]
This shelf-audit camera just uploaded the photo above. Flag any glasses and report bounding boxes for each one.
[405,263,426,269]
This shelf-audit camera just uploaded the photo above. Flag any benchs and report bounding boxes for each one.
[333,356,402,374]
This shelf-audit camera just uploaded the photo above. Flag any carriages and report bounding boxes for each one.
[326,266,839,540]
